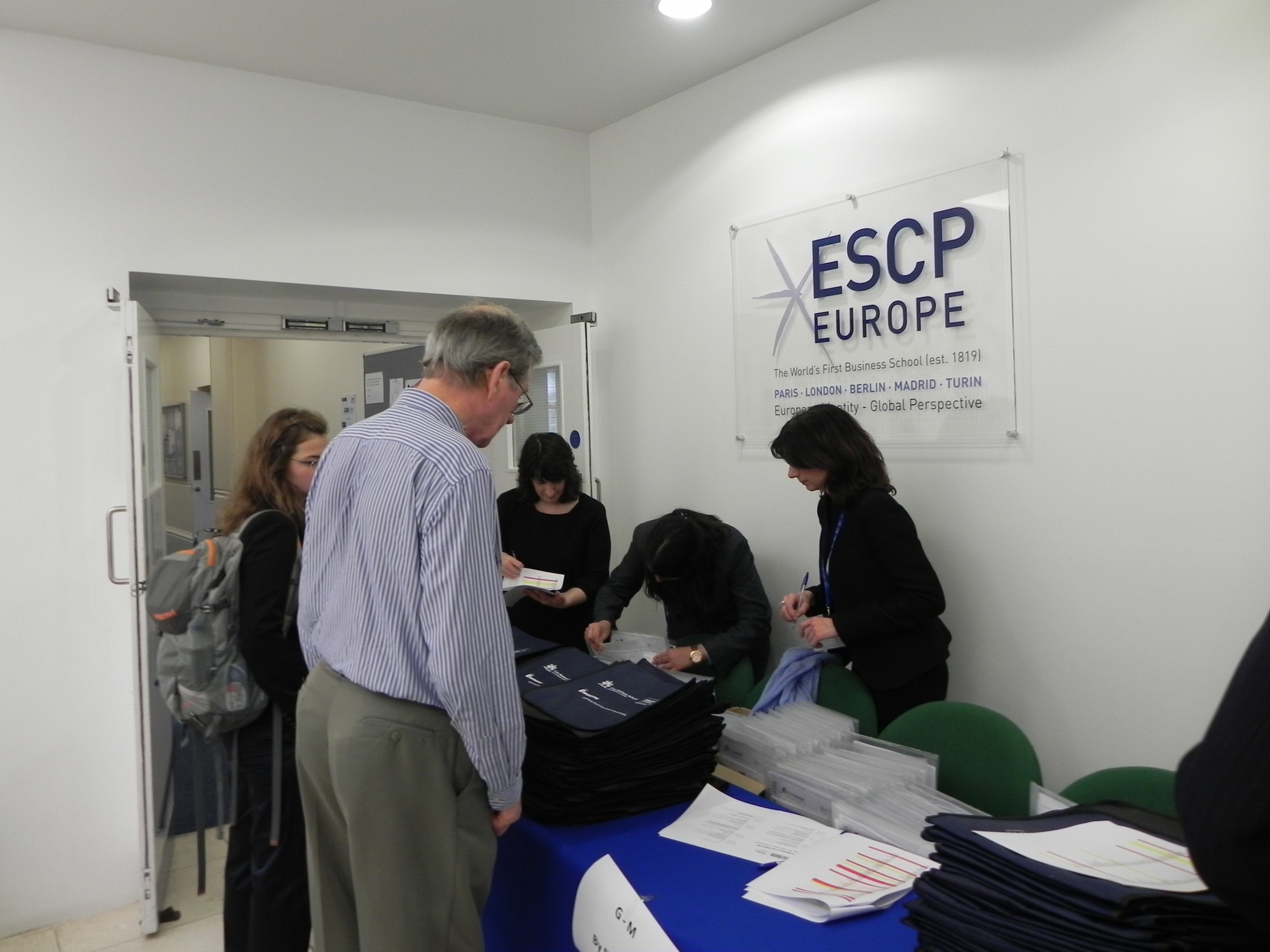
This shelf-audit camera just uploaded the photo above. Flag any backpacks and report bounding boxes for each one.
[144,508,303,733]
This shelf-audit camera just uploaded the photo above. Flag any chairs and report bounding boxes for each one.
[677,633,752,722]
[1059,765,1182,819]
[746,662,878,738]
[874,699,1047,822]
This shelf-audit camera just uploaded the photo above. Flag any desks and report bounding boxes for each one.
[477,764,931,952]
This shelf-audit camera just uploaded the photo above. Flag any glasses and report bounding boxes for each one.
[290,458,319,468]
[488,366,533,416]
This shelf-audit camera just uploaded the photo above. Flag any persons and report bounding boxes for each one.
[580,507,774,714]
[771,401,953,739]
[295,304,544,952]
[208,404,333,951]
[496,433,616,651]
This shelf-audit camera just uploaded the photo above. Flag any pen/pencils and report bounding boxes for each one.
[792,572,810,629]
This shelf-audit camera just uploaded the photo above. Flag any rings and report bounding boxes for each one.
[805,628,810,635]
[777,601,786,608]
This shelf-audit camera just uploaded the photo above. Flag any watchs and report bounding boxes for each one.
[689,644,703,666]
